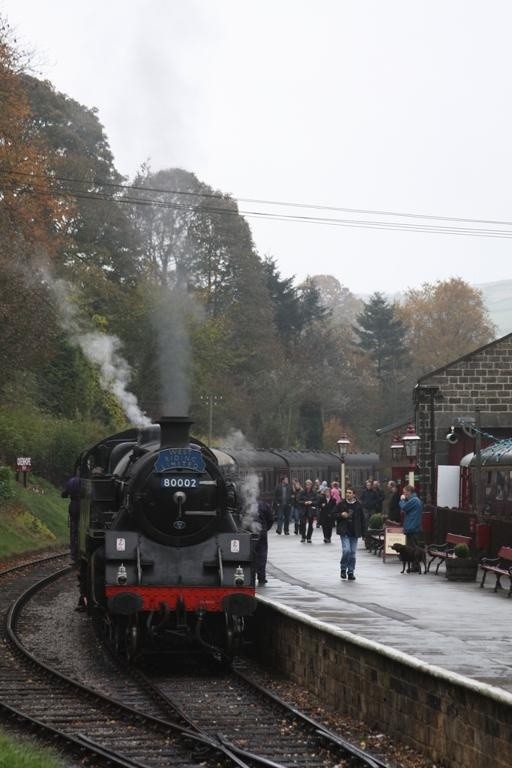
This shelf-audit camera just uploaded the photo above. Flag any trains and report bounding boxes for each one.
[64,416,380,671]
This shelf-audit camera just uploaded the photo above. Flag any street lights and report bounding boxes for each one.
[336,433,350,500]
[402,416,420,487]
[391,435,404,463]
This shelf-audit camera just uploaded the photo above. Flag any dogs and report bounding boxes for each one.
[391,543,427,574]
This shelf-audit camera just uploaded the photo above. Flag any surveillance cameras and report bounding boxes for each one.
[446,426,458,443]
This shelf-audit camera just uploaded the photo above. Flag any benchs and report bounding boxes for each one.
[363,518,511,602]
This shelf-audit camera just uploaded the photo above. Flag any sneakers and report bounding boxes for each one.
[348,573,355,579]
[276,529,331,543]
[341,570,346,578]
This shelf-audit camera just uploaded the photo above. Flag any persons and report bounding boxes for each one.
[398,485,423,573]
[331,486,367,581]
[275,475,400,544]
[243,488,274,585]
